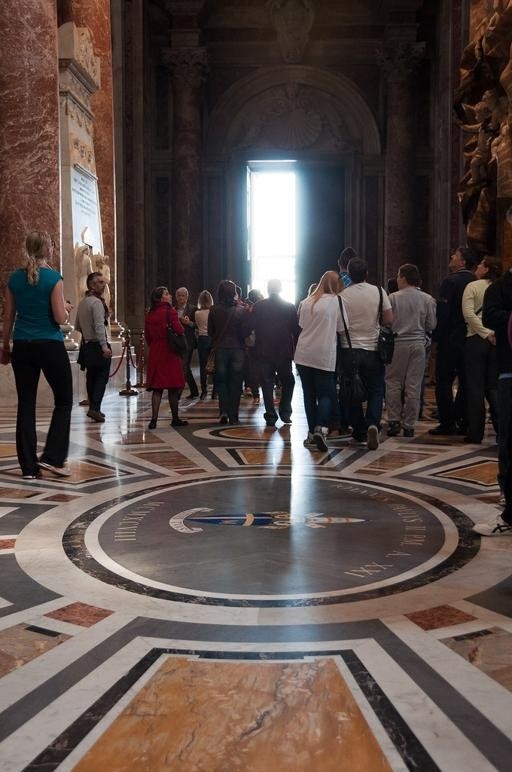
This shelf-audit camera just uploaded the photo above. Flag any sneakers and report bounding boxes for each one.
[245,387,252,396]
[472,513,511,536]
[253,397,260,404]
[36,459,72,477]
[280,416,292,423]
[367,425,379,450]
[220,415,239,425]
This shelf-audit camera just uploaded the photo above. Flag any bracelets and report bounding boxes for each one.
[0,346,10,352]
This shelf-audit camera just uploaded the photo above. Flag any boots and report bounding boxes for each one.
[304,425,328,452]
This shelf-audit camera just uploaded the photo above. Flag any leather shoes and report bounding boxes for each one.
[87,409,105,422]
[171,420,188,427]
[386,422,414,437]
[149,419,157,429]
[428,428,457,434]
[186,390,218,399]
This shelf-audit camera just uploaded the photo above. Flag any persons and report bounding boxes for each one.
[0,231,74,481]
[144,279,299,428]
[75,245,94,302]
[293,247,503,451]
[471,263,512,537]
[95,255,111,309]
[74,270,115,423]
[456,0,512,186]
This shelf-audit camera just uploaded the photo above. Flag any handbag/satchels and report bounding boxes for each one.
[168,328,186,354]
[205,348,216,375]
[378,329,394,364]
[340,368,368,402]
